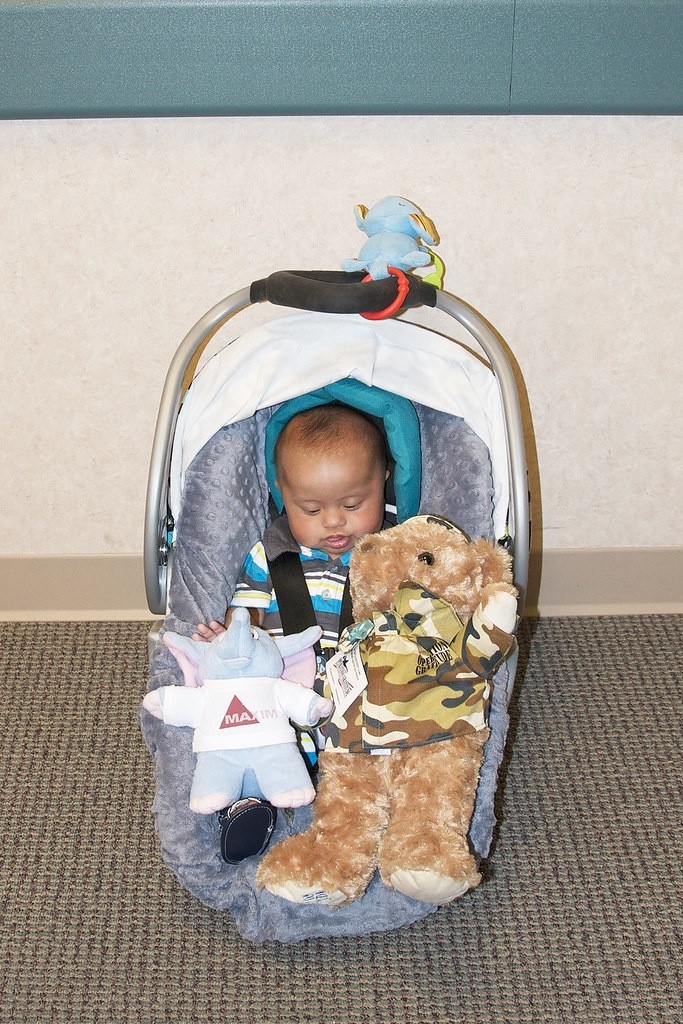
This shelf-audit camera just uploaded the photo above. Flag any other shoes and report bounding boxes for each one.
[220,798,277,864]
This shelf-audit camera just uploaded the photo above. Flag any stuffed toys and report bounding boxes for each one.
[257,512,518,903]
[342,193,434,278]
[140,606,334,813]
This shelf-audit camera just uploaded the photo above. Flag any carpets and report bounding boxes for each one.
[1,615,682,1017]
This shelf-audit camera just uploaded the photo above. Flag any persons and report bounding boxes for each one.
[191,404,405,866]
